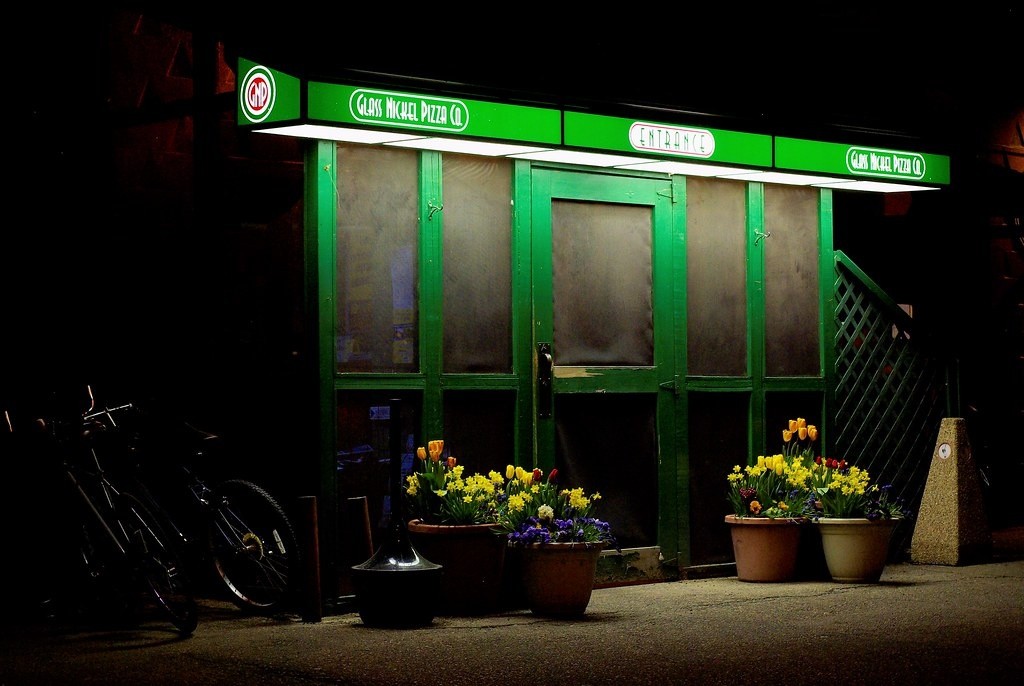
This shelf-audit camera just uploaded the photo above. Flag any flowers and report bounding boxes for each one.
[398,439,623,555]
[722,416,915,523]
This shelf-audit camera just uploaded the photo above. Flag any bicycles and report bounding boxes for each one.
[6,383,303,638]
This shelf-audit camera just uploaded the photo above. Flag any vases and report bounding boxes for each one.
[724,514,808,582]
[811,517,902,583]
[515,541,606,616]
[408,516,509,613]
